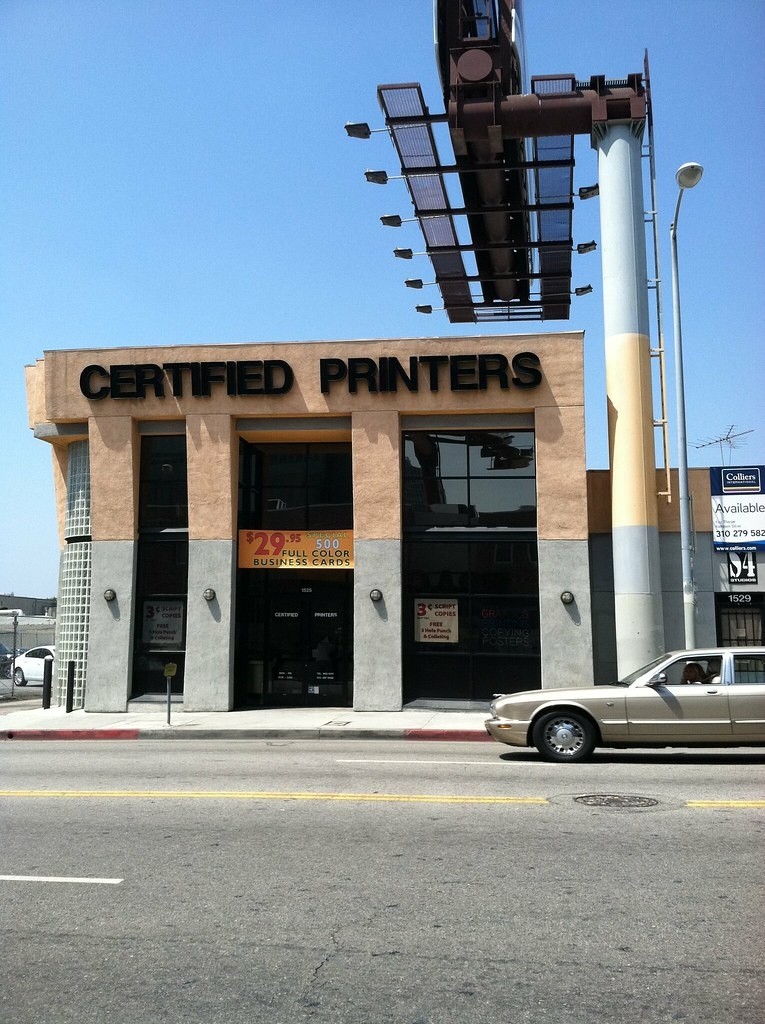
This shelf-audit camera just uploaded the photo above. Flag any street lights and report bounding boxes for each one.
[671,161,703,651]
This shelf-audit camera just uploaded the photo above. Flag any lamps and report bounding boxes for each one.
[379,215,447,227]
[573,183,599,200]
[572,239,597,255]
[364,168,438,184]
[344,121,426,139]
[561,591,574,604]
[415,304,464,314]
[203,589,216,601]
[370,589,383,601]
[103,589,116,601]
[392,247,455,259]
[404,279,463,289]
[571,284,593,297]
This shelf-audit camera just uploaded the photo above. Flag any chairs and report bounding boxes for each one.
[707,662,721,683]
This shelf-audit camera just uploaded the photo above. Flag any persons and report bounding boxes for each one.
[680,663,706,685]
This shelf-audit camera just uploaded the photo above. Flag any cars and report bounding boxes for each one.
[484,646,765,764]
[0,646,55,686]
